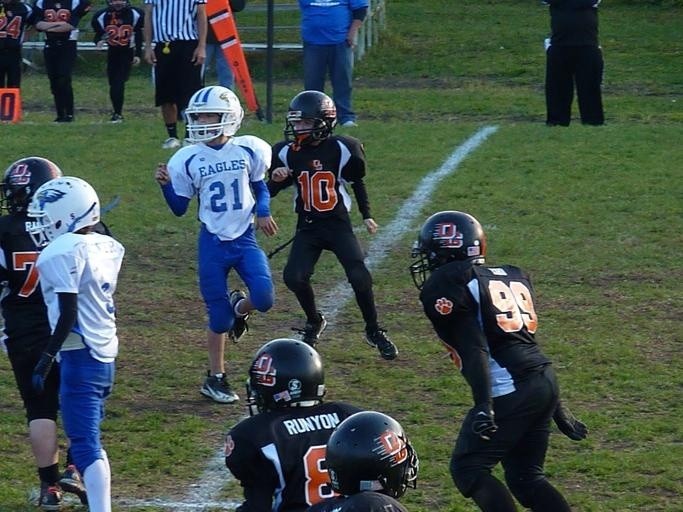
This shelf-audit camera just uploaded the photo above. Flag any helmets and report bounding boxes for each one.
[24,176,101,248]
[283,90,338,148]
[245,337,327,418]
[324,410,421,500]
[0,156,64,217]
[408,210,487,292]
[184,85,245,144]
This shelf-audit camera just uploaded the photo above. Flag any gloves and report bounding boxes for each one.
[468,401,500,441]
[31,352,56,391]
[554,402,587,441]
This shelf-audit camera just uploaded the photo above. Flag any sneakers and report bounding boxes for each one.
[198,371,239,405]
[37,481,64,506]
[340,120,359,129]
[363,326,400,361]
[162,135,180,150]
[300,312,327,347]
[62,114,76,123]
[109,113,124,123]
[227,289,249,344]
[58,466,88,505]
[52,117,63,124]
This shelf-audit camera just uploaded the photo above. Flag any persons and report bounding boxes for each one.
[305,411,418,512]
[0,0,43,88]
[544,0,604,125]
[0,157,88,512]
[299,0,370,125]
[33,0,92,123]
[27,177,125,512]
[411,213,586,512]
[205,0,248,91]
[267,91,398,361]
[143,0,207,148]
[91,0,145,122]
[224,339,363,512]
[155,86,278,401]
